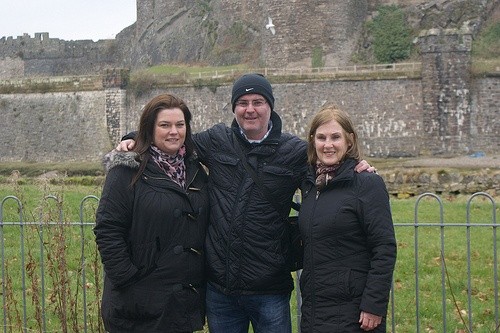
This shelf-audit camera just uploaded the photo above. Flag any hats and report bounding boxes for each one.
[230,72,275,113]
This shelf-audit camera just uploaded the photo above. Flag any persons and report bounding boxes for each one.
[93,94,212,333]
[108,73,378,333]
[298,108,398,333]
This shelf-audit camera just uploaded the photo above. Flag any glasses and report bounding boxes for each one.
[235,100,267,108]
